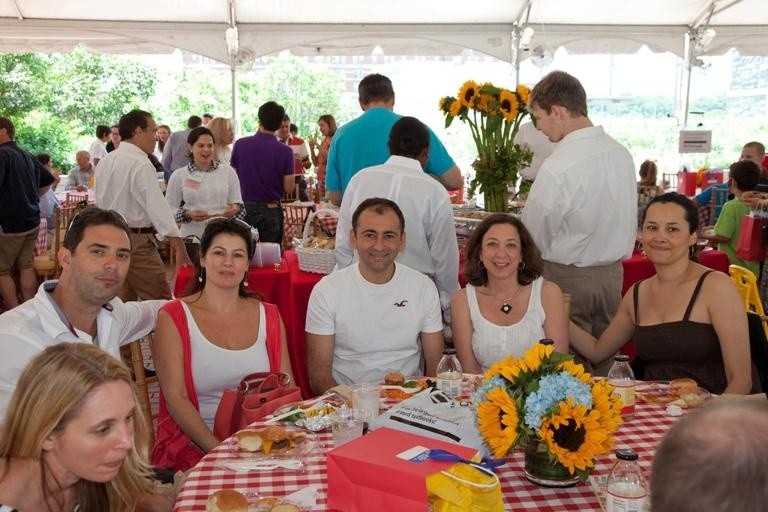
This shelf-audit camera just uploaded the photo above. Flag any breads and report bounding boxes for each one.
[384,371,405,385]
[262,427,292,456]
[204,490,249,512]
[268,503,299,512]
[235,431,263,454]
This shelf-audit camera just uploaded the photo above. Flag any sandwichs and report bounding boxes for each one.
[670,378,697,395]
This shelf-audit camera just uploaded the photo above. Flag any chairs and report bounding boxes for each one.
[131,328,162,451]
[637,184,660,211]
[308,173,323,202]
[280,182,318,239]
[66,191,89,227]
[31,202,79,291]
[662,171,679,191]
[709,185,733,227]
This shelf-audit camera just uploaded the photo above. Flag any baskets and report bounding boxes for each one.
[292,207,343,277]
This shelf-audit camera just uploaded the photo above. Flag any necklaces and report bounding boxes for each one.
[486,287,521,315]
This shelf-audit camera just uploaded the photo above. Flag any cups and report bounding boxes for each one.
[331,408,373,447]
[348,383,381,421]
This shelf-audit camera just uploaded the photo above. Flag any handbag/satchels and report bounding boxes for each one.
[214,369,301,439]
[737,204,767,263]
[324,426,500,512]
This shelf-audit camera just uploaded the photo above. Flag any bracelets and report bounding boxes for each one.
[52,185,56,188]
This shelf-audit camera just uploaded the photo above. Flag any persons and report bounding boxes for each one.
[304,197,446,396]
[106,125,122,153]
[565,191,753,396]
[165,127,246,243]
[741,141,768,192]
[741,190,768,210]
[520,70,639,376]
[635,160,663,228]
[275,114,311,204]
[90,125,113,166]
[230,101,296,244]
[324,74,463,207]
[203,114,213,126]
[93,109,192,381]
[692,165,732,225]
[697,160,761,279]
[162,115,202,183]
[290,124,297,137]
[64,150,95,191]
[649,395,768,512]
[307,114,337,196]
[36,154,61,219]
[152,217,304,473]
[334,116,462,339]
[450,214,569,374]
[157,124,171,153]
[0,116,56,310]
[0,204,174,428]
[208,117,234,164]
[0,342,175,512]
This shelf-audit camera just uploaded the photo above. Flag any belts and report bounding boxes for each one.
[249,200,281,208]
[131,226,156,234]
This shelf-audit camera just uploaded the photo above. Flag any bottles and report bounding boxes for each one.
[435,349,463,395]
[608,355,636,422]
[606,450,647,512]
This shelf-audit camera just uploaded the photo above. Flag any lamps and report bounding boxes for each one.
[694,28,717,55]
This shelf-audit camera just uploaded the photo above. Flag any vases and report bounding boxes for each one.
[523,436,590,488]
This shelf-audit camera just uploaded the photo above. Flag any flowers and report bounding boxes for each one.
[473,342,625,481]
[439,80,536,213]
